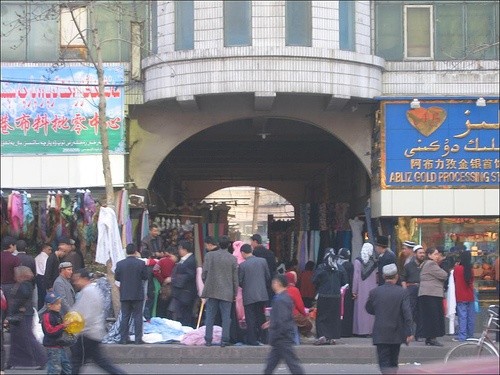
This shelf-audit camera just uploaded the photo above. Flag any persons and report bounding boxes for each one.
[365,263,414,375]
[261,274,306,375]
[39,292,72,375]
[0,223,500,371]
[68,269,128,375]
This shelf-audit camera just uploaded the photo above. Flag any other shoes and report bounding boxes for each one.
[314,336,335,345]
[221,340,230,347]
[256,340,264,346]
[205,340,211,346]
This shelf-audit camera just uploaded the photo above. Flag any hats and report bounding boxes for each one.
[404,240,415,247]
[437,246,447,252]
[45,292,62,303]
[58,262,72,269]
[413,245,422,252]
[374,235,388,247]
[383,263,398,276]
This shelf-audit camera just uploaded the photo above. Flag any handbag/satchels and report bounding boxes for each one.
[55,333,77,346]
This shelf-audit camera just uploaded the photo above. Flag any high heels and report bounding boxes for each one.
[425,338,440,346]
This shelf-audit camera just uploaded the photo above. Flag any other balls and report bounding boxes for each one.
[63,311,83,334]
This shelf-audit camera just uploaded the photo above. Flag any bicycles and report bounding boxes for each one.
[444,304,499,363]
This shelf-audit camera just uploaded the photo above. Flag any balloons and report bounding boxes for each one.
[64,311,85,334]
